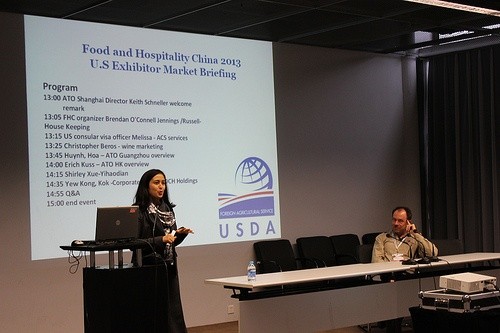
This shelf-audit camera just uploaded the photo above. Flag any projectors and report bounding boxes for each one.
[439,272,497,294]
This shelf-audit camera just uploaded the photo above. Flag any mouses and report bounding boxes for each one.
[71,240,84,246]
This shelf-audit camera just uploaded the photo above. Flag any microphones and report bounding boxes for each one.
[386,229,439,265]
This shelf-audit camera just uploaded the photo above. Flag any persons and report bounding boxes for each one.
[372,206,438,263]
[130,170,192,333]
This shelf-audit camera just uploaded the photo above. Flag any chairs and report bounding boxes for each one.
[295,235,359,288]
[252,239,320,295]
[361,231,401,282]
[329,232,374,286]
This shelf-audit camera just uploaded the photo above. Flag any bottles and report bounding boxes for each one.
[247,261,256,283]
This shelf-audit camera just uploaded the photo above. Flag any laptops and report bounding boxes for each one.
[82,206,139,245]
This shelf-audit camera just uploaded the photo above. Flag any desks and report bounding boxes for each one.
[203,252,500,333]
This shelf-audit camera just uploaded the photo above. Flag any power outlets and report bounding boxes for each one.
[228,305,235,314]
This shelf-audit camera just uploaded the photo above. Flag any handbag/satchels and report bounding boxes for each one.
[418,289,500,314]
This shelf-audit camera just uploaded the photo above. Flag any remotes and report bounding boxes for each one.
[167,230,176,246]
